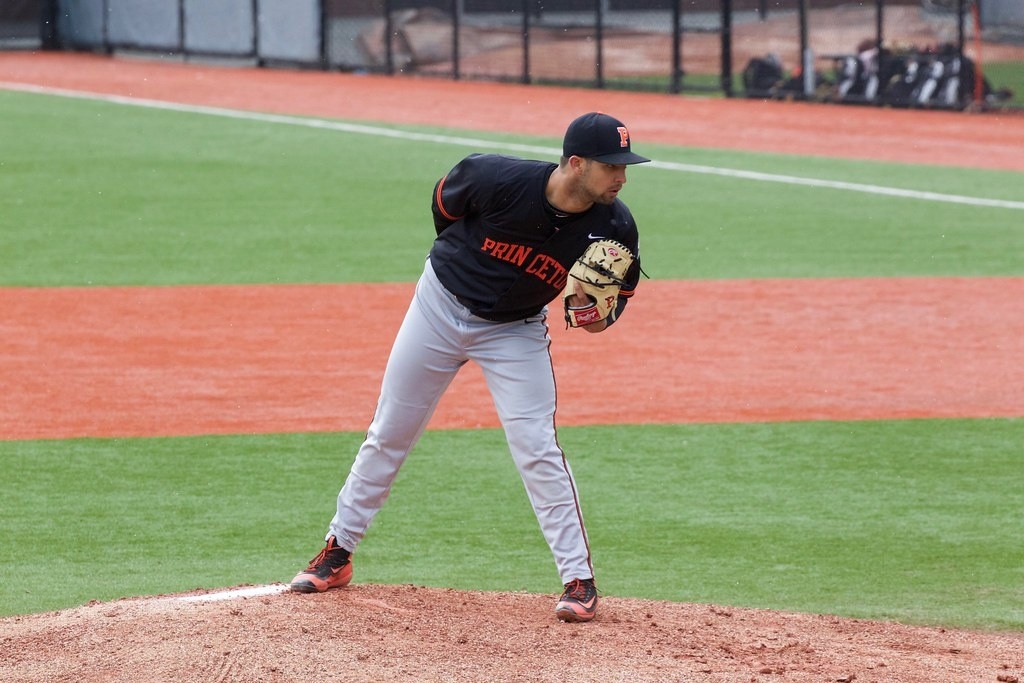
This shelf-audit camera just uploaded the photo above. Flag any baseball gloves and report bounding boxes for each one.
[559,237,636,329]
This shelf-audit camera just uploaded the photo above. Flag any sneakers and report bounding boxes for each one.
[291,535,353,591]
[555,578,600,621]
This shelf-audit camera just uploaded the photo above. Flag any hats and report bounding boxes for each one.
[563,112,650,165]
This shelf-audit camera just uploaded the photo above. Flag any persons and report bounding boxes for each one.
[290,111,651,622]
